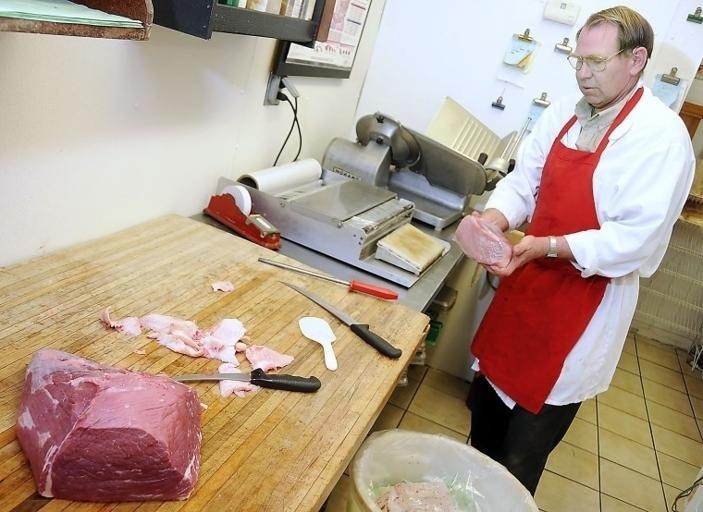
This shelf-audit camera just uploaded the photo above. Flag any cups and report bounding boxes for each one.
[566,46,636,75]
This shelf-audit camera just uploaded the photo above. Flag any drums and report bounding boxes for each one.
[276,279,403,359]
[174,368,324,396]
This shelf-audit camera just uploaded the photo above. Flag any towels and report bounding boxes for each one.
[350,430,539,512]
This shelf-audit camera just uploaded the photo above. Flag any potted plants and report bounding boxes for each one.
[274,1,372,79]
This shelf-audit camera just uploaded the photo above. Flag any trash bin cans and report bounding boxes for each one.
[545,234,559,260]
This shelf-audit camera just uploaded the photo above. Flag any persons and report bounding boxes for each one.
[463,5,696,498]
